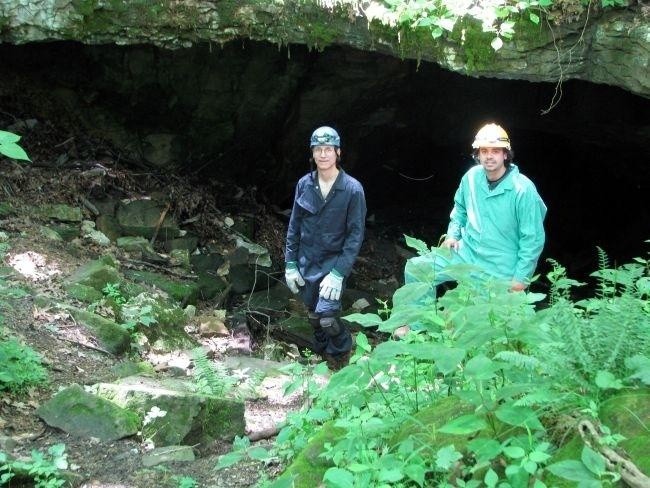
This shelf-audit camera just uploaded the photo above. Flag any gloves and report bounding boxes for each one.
[318,267,345,300]
[284,261,305,294]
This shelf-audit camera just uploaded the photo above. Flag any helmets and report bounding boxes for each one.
[309,124,342,148]
[471,122,512,151]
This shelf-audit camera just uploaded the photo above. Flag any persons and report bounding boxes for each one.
[281,124,368,373]
[394,123,549,339]
